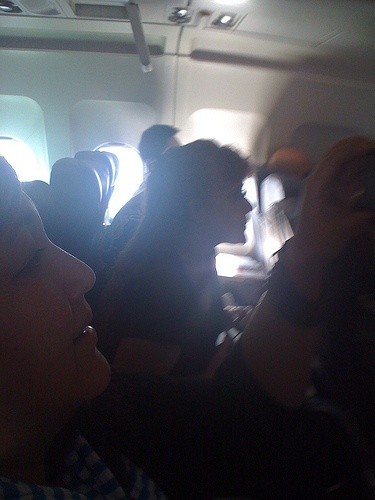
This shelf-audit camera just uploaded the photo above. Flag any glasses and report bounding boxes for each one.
[233,191,248,205]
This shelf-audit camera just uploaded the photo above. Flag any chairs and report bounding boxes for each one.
[25,145,375,500]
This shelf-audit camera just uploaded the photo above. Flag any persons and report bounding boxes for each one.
[96,137,375,500]
[98,125,180,284]
[191,127,375,464]
[0,156,197,500]
